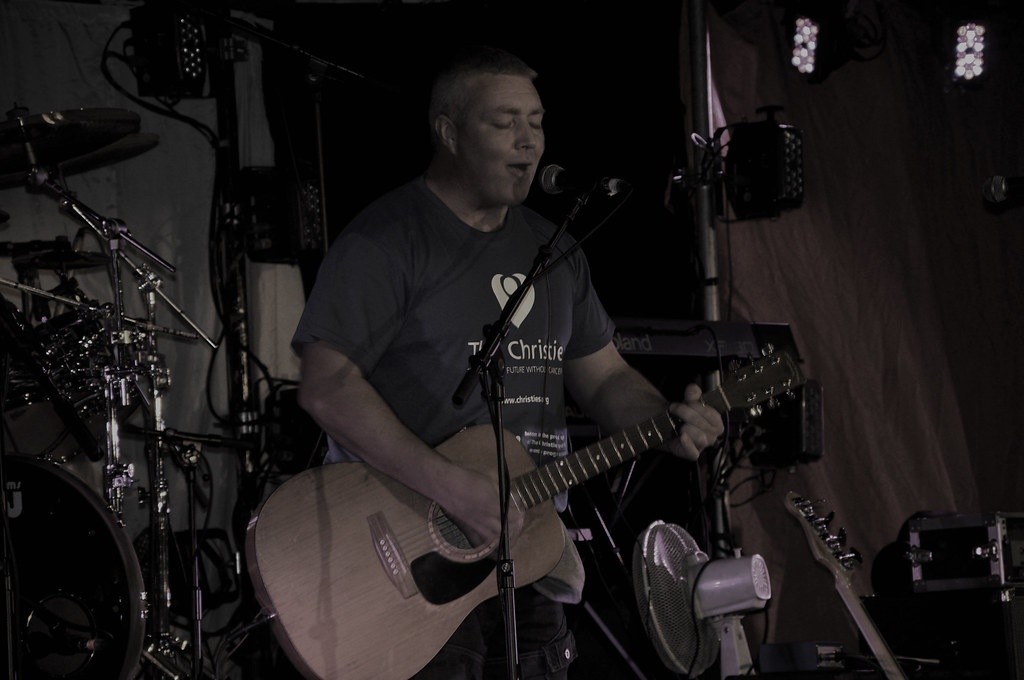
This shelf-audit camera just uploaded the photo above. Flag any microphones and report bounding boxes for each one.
[57,631,104,656]
[66,227,85,281]
[542,166,632,194]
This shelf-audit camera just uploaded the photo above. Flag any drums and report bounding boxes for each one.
[0,331,77,455]
[35,311,142,446]
[0,446,148,679]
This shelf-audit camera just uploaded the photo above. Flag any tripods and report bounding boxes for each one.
[56,170,218,680]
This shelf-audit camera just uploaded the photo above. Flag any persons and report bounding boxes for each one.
[290,47,727,680]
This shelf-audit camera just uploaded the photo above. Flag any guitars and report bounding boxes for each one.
[244,344,805,680]
[783,489,904,680]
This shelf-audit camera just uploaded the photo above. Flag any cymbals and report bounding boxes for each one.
[10,251,112,271]
[0,108,143,178]
[0,132,159,188]
